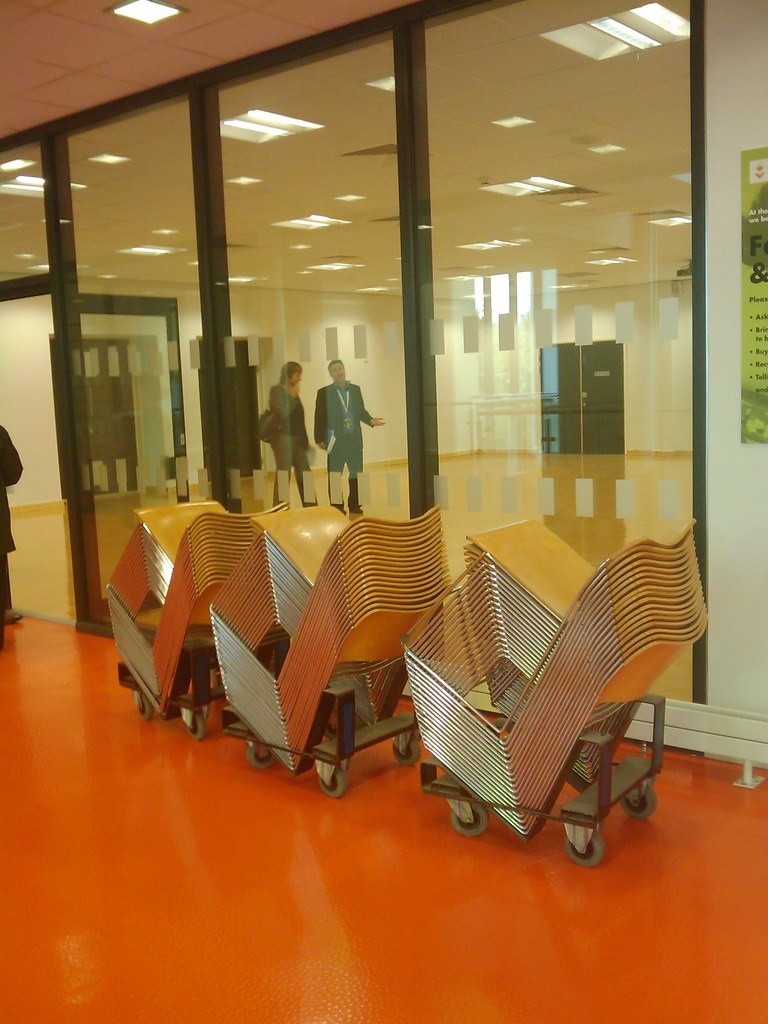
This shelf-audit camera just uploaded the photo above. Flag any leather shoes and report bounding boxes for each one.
[4,614,23,623]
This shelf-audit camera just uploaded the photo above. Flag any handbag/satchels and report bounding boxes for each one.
[255,410,278,444]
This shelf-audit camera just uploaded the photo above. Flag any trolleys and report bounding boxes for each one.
[117,637,218,740]
[218,663,422,799]
[416,692,670,868]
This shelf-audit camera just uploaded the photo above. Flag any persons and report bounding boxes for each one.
[315,359,386,515]
[269,361,320,511]
[0,426,24,625]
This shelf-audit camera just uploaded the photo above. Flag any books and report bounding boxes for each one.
[324,428,336,455]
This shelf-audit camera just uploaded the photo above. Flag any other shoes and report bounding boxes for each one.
[341,510,347,515]
[349,505,363,514]
